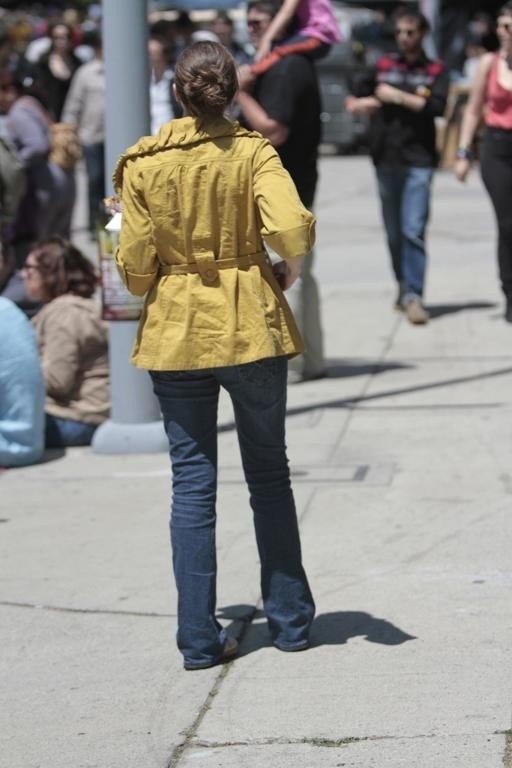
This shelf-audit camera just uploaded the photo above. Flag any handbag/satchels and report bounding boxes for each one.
[48,120,83,169]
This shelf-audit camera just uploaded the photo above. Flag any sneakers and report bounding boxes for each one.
[222,634,240,660]
[396,281,428,323]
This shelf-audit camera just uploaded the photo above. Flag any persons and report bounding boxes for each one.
[113,42,316,671]
[343,1,512,328]
[1,0,341,469]
